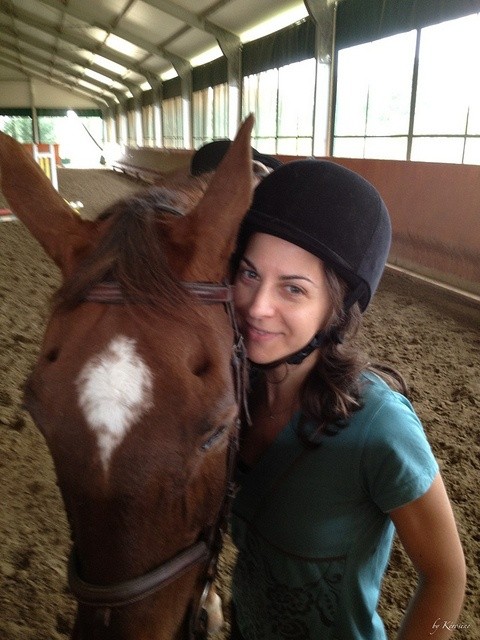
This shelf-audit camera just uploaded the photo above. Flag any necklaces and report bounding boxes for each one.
[257,391,296,419]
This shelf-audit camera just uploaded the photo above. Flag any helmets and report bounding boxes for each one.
[233,161,393,315]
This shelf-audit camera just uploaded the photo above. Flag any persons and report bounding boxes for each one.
[221,159,468,640]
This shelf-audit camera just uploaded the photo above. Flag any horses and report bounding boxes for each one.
[1,112,275,640]
